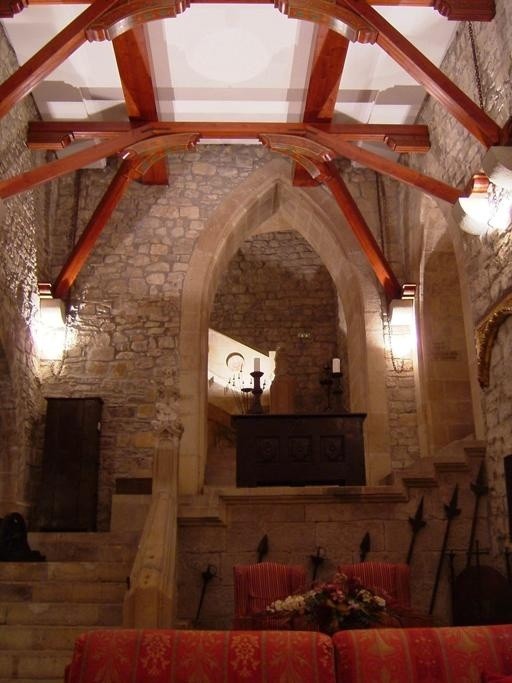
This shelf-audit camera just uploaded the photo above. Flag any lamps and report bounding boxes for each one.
[40,298,65,327]
[458,197,492,222]
[296,324,312,345]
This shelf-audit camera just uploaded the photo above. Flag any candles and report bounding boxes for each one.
[254,358,260,372]
[332,357,341,373]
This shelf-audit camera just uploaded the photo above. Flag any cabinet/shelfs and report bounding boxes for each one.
[38,396,105,532]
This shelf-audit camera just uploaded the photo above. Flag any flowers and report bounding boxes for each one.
[265,571,389,634]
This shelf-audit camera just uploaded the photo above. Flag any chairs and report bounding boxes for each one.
[336,562,429,626]
[234,562,308,630]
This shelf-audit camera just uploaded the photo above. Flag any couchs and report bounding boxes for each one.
[332,624,511,683]
[62,628,337,683]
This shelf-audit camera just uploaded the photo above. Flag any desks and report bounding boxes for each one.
[231,412,367,486]
